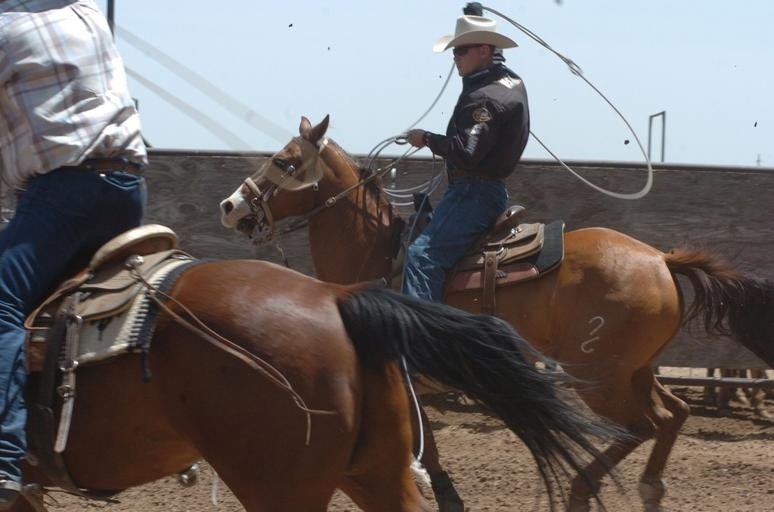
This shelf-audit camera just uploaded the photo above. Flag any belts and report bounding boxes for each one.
[88,155,139,174]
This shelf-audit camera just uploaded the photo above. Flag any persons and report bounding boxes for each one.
[402,3,529,382]
[1,0,149,509]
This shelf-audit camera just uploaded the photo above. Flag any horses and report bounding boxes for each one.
[219,114,774,512]
[29,259,644,512]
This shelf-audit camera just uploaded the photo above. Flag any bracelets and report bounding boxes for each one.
[423,133,432,147]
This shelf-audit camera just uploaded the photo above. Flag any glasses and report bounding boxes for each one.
[452,42,483,55]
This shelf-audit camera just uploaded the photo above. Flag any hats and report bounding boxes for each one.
[434,15,518,53]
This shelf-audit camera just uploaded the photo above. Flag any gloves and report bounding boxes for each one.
[463,2,482,15]
